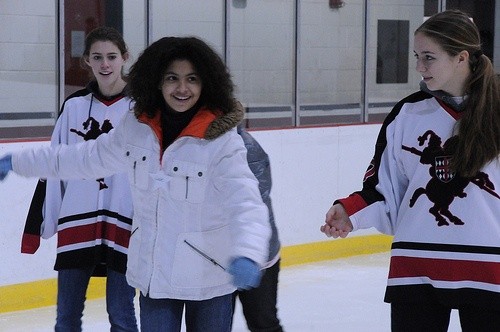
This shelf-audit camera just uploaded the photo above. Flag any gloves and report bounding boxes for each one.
[0,154,13,182]
[225,257,262,290]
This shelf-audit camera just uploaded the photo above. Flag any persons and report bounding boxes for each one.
[0,36,271,332]
[20,26,139,332]
[319,9,500,332]
[230,124,284,332]
